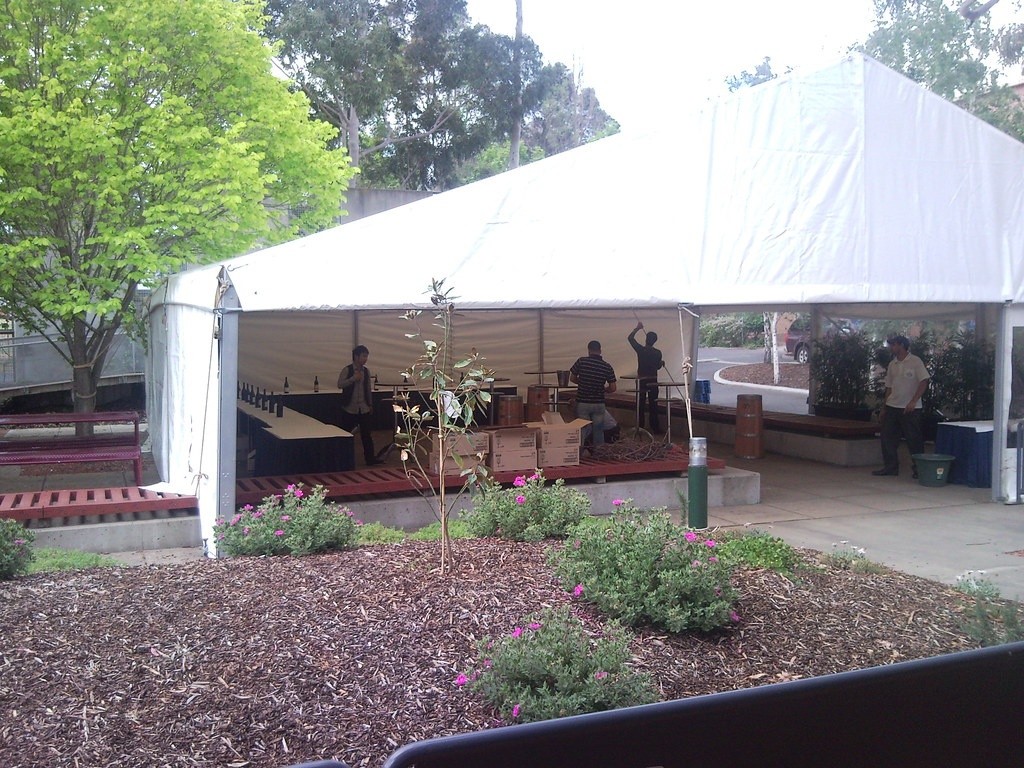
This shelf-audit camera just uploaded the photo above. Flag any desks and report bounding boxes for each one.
[259,385,519,428]
[536,383,579,411]
[934,419,1024,487]
[654,381,689,443]
[236,395,353,475]
[0,411,140,446]
[619,373,654,441]
[374,382,415,462]
[475,377,511,425]
[523,370,559,384]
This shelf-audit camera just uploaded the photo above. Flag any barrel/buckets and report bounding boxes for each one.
[912,453,957,486]
[695,379,711,404]
[525,385,550,422]
[557,370,570,386]
[735,395,761,459]
[498,394,524,426]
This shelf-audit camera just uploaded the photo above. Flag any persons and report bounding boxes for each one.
[870,337,932,478]
[567,402,621,454]
[337,345,385,465]
[627,321,666,433]
[570,341,617,445]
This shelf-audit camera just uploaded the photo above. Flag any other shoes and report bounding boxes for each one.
[913,472,918,478]
[366,456,384,466]
[872,468,898,475]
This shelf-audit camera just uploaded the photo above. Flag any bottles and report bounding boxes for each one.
[238,381,241,399]
[250,385,255,404]
[284,377,289,393]
[277,394,283,417]
[255,387,261,407]
[459,372,466,384]
[374,374,378,390]
[245,384,250,402]
[269,391,275,412]
[241,382,246,400]
[262,389,268,410]
[314,376,319,392]
[403,376,408,390]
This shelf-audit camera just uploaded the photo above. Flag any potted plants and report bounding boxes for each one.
[802,326,1024,441]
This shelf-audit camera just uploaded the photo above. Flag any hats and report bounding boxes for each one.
[887,336,909,345]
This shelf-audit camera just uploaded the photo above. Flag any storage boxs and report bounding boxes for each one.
[247,448,255,475]
[695,379,712,404]
[430,410,592,475]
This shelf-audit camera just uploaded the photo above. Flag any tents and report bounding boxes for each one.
[137,54,1024,560]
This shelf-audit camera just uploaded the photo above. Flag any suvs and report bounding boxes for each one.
[784,314,858,365]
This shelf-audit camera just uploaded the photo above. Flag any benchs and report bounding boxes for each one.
[0,437,142,486]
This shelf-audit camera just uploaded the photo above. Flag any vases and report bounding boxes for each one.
[912,453,955,487]
